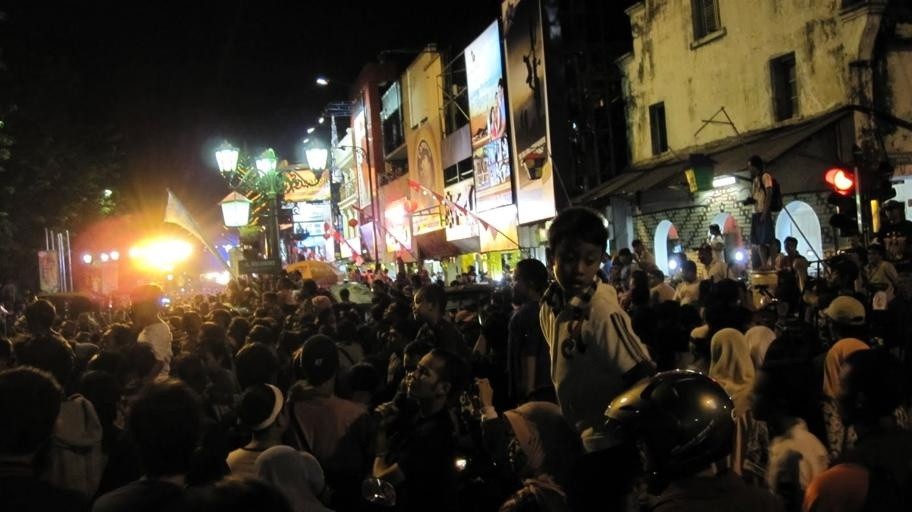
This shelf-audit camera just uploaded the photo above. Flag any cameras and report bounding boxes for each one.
[743,198,755,205]
[467,386,480,412]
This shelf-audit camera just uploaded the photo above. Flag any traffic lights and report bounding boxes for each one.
[825,165,863,237]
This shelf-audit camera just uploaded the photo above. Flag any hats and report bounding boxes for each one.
[821,296,867,329]
[233,381,287,432]
[300,334,341,387]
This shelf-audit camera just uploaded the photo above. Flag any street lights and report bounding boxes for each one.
[213,137,328,280]
[315,74,379,273]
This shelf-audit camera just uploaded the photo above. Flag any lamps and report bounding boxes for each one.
[712,175,737,188]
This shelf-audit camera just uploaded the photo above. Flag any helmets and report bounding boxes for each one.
[602,365,744,495]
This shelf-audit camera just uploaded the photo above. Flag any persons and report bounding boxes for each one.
[480,76,510,183]
[744,154,780,272]
[415,143,435,194]
[1,198,912,512]
[379,164,410,188]
